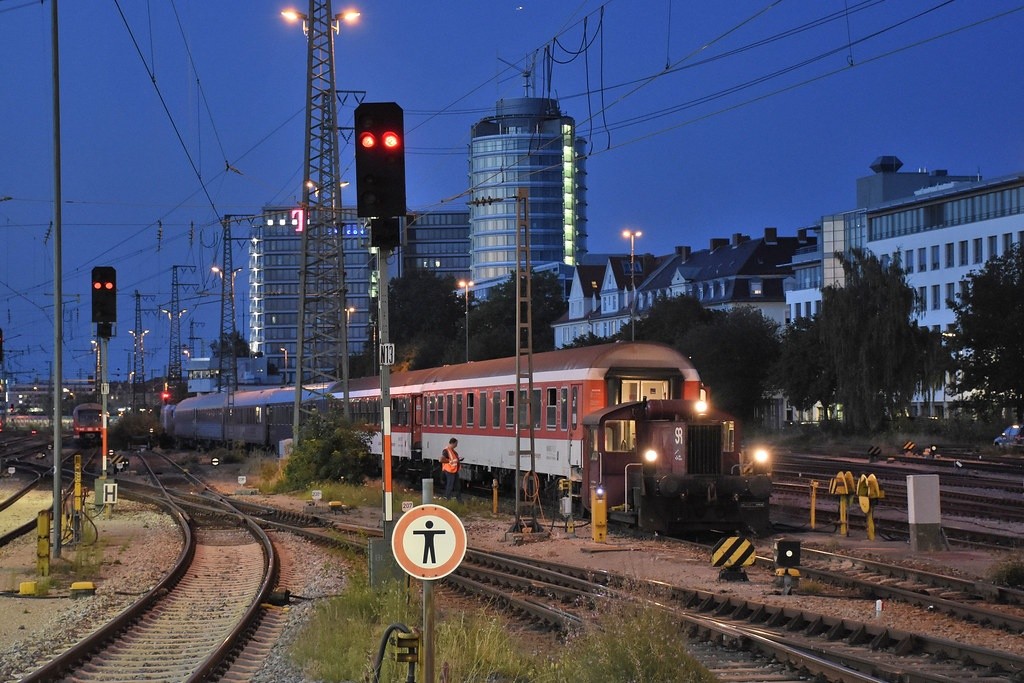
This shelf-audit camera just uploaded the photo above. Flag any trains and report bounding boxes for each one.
[71,402,110,450]
[173,339,774,539]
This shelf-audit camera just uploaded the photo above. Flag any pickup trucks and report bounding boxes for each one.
[992,424,1024,452]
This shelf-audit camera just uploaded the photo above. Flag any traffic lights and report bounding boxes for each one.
[163,391,171,405]
[351,98,411,222]
[88,265,120,324]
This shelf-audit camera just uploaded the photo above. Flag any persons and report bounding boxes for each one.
[439,438,465,504]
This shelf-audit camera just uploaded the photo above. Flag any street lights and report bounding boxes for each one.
[306,179,352,382]
[273,1,363,455]
[624,228,643,345]
[211,266,244,393]
[91,339,105,407]
[161,309,187,381]
[127,329,151,413]
